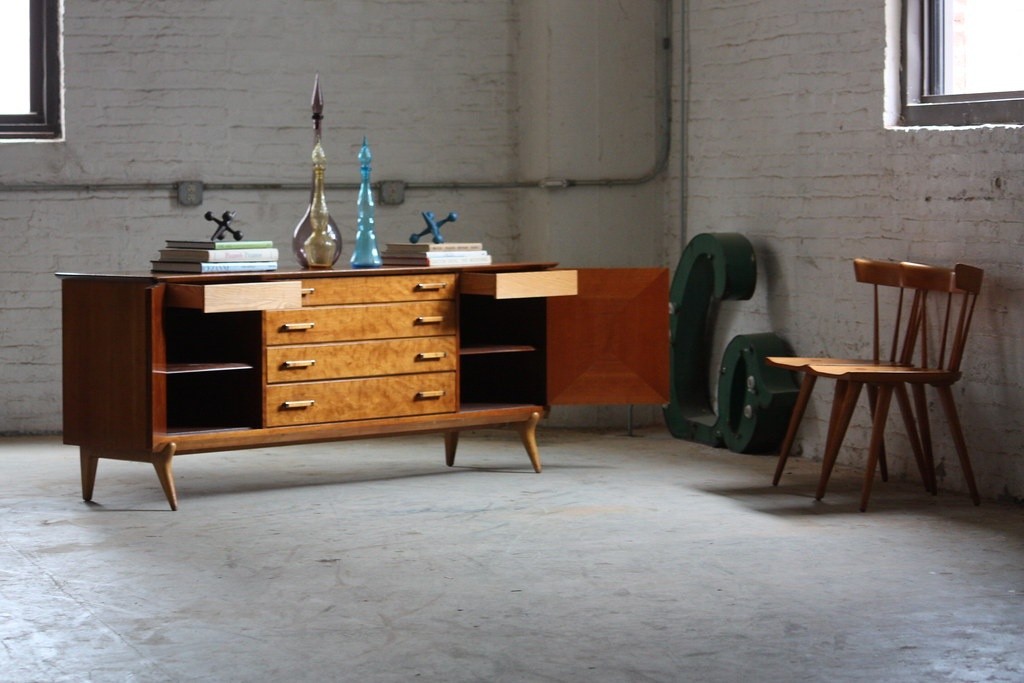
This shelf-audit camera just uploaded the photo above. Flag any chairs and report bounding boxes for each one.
[765,257,984,512]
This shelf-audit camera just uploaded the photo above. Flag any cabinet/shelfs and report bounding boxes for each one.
[56,262,671,513]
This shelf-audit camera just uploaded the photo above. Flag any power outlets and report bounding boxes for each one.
[380,180,405,205]
[177,181,203,205]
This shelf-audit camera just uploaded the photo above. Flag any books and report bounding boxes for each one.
[380,243,492,266]
[150,240,279,273]
